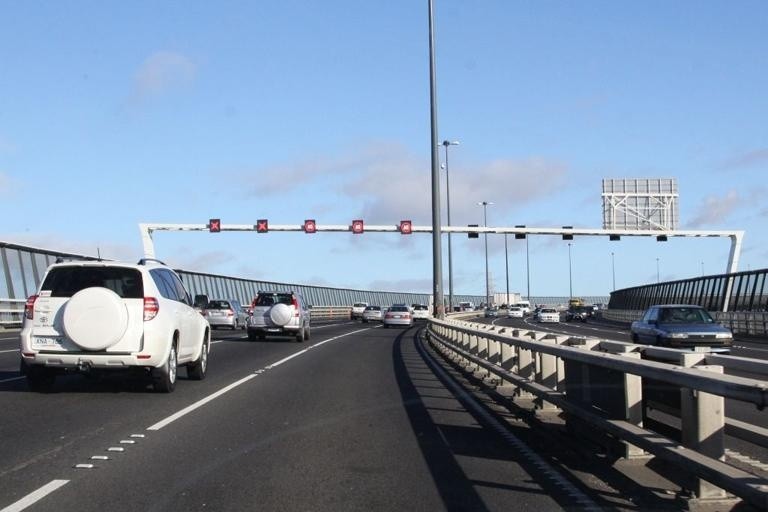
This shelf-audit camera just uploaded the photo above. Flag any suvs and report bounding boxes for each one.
[246,289,313,343]
[18,255,214,394]
[350,302,369,320]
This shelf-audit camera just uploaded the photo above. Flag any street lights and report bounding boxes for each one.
[655,258,659,284]
[610,253,614,293]
[477,201,494,311]
[567,242,573,300]
[437,140,461,310]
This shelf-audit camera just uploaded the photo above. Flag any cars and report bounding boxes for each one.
[362,305,384,323]
[443,298,609,324]
[383,306,414,329]
[630,303,734,356]
[205,299,248,331]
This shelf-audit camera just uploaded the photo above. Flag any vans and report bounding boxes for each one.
[411,303,432,321]
